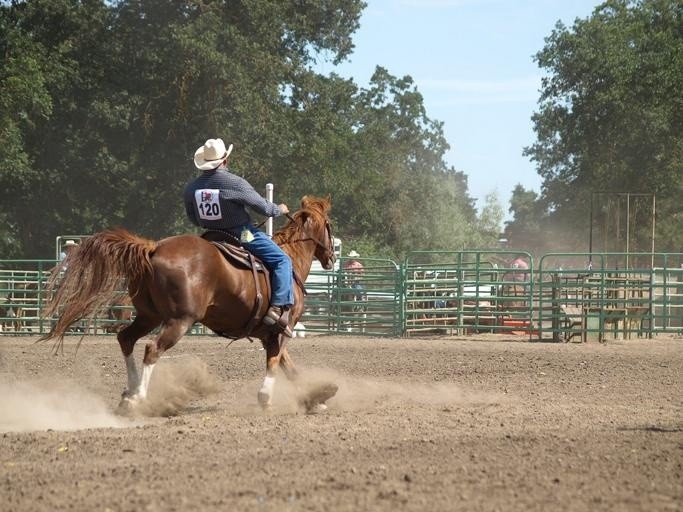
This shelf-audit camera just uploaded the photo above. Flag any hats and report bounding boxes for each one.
[61,240,79,249]
[193,137,234,171]
[347,250,361,257]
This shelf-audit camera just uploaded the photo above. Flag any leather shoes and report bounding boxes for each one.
[262,305,294,338]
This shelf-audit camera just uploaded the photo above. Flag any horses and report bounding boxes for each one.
[0,269,534,338]
[31,193,337,417]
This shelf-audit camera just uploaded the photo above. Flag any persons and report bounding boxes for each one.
[492,252,531,288]
[184,138,295,338]
[58,240,78,272]
[343,250,365,312]
